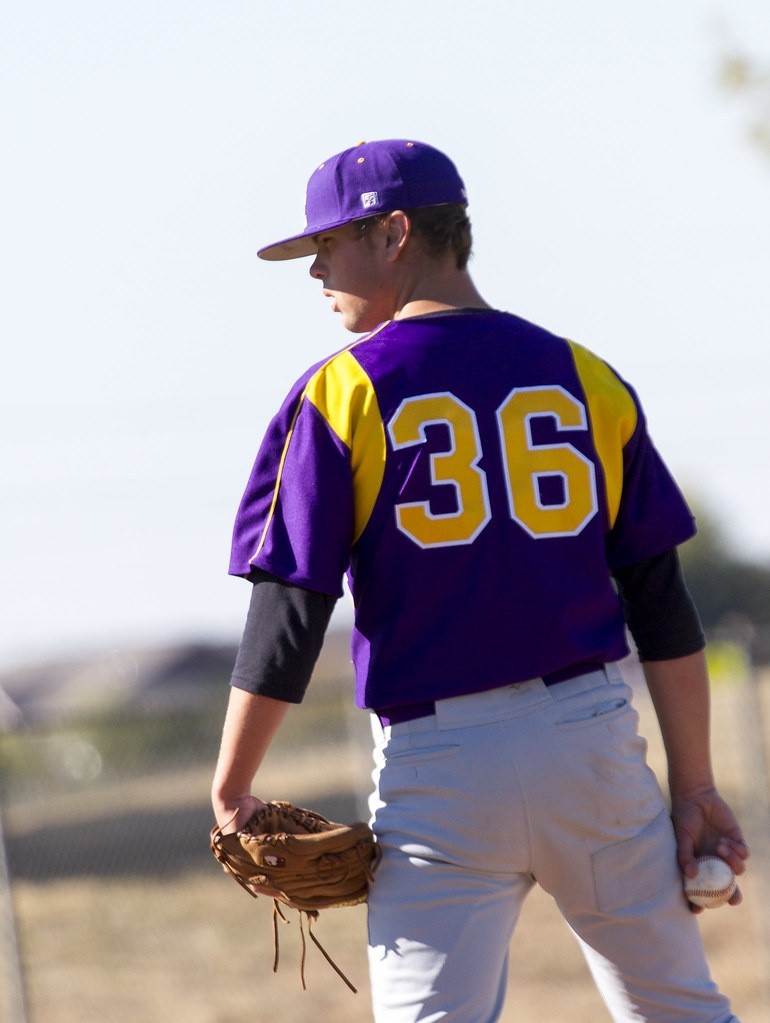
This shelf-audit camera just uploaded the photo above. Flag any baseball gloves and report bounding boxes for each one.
[209,800,380,996]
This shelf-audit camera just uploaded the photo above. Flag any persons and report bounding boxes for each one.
[209,141,747,1022]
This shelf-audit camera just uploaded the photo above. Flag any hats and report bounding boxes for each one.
[258,140,469,261]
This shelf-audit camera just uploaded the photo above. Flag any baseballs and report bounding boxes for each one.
[684,856,738,908]
